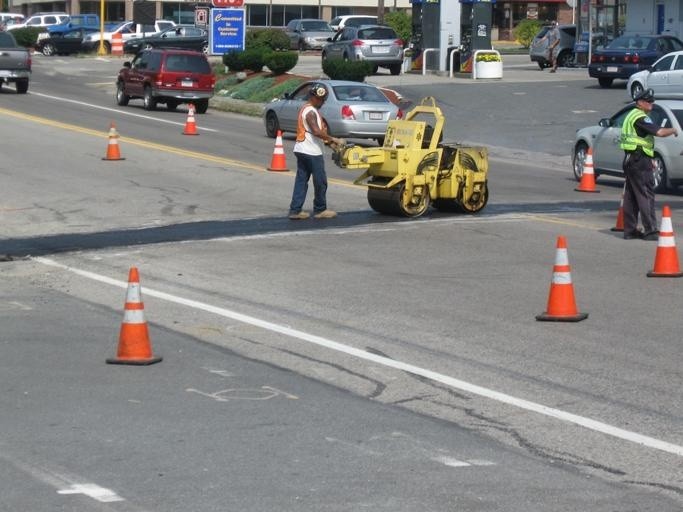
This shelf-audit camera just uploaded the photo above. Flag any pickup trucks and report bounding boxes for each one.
[0,31,31,94]
[47,13,132,38]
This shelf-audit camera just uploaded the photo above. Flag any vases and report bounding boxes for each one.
[476,61,502,79]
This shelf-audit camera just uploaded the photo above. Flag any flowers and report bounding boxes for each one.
[475,55,501,62]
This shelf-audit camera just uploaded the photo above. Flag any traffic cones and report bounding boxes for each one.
[611,176,643,233]
[104,267,163,365]
[573,146,602,194]
[535,234,589,322]
[179,103,199,136]
[265,129,291,173]
[644,204,683,278]
[100,122,125,161]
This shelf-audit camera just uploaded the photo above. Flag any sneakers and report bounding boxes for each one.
[288,210,310,219]
[313,209,338,219]
[624,230,660,241]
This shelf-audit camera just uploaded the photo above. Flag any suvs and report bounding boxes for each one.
[6,12,70,31]
[114,48,215,114]
[321,26,404,76]
[82,20,175,51]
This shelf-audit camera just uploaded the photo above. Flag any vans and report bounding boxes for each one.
[0,14,23,28]
[329,15,378,31]
[529,24,578,70]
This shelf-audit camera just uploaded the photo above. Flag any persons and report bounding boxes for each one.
[538,21,561,73]
[619,88,678,240]
[287,82,342,220]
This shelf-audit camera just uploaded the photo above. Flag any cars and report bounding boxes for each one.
[577,27,613,52]
[261,80,402,146]
[124,26,208,54]
[35,27,110,56]
[284,19,339,51]
[588,35,683,87]
[572,99,682,193]
[626,50,683,102]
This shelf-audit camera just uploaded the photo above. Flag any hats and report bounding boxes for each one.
[633,87,656,101]
[308,82,330,101]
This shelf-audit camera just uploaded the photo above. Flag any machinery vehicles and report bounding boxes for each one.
[332,96,489,219]
